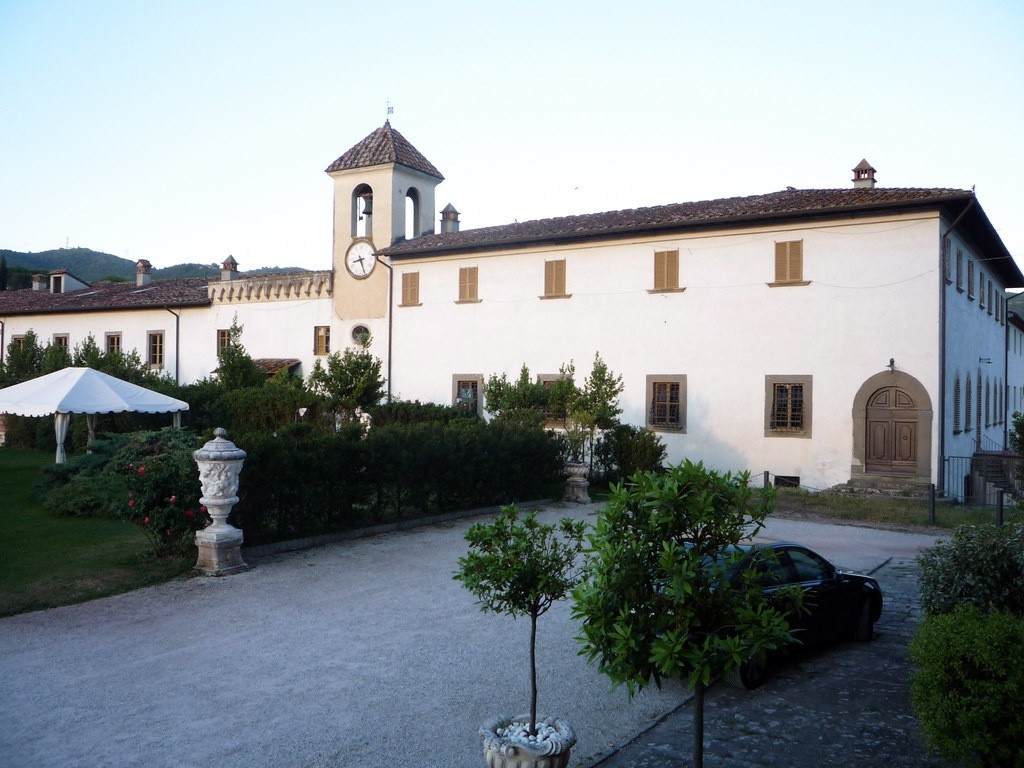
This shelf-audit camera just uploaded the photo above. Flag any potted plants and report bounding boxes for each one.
[451,504,587,768]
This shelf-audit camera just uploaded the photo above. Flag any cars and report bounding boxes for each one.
[683,539,885,691]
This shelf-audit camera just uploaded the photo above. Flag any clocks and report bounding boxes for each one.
[344,238,378,280]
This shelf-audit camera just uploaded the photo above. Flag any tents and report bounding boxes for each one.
[0,367,189,464]
